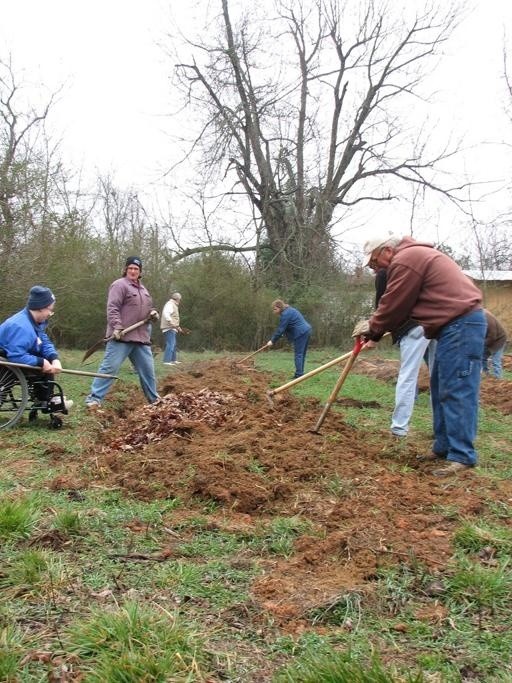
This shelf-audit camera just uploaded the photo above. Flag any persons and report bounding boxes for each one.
[352,234,487,477]
[159,292,182,366]
[482,308,507,379]
[266,298,311,379]
[85,256,159,410]
[360,262,438,438]
[0,286,74,416]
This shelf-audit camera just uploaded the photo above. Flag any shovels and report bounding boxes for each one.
[81,315,155,368]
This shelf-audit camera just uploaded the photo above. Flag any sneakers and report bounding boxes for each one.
[50,396,73,413]
[417,451,446,460]
[434,462,472,475]
[162,361,181,365]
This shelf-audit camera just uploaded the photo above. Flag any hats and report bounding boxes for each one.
[127,256,142,268]
[27,285,55,309]
[362,230,397,268]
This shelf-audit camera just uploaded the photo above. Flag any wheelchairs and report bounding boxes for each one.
[0,356,69,431]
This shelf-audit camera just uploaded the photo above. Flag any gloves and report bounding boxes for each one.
[113,329,124,340]
[150,310,160,323]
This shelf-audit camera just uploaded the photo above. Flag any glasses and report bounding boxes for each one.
[370,257,376,269]
[45,307,55,316]
